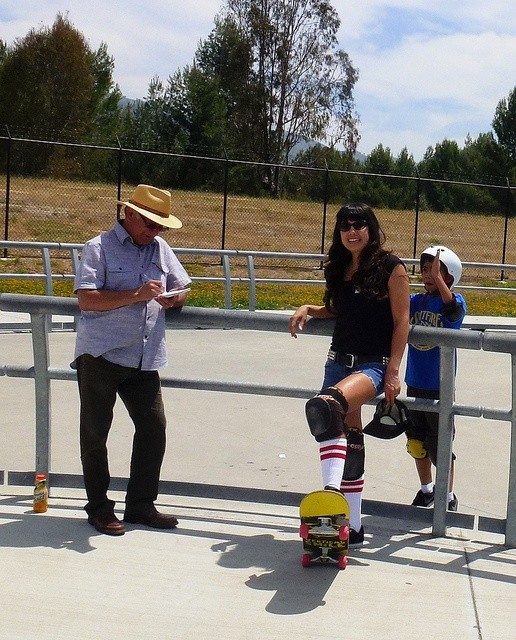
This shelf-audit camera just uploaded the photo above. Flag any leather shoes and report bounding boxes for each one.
[88,510,126,535]
[124,503,178,528]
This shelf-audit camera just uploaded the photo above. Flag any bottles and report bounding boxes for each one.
[33,475,49,513]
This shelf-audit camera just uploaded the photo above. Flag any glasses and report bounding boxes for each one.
[139,213,169,232]
[336,220,368,232]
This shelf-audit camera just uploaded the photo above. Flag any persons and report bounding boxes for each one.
[404,244,467,512]
[289,203,411,550]
[70,185,191,537]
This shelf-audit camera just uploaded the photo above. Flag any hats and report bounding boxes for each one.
[117,184,183,229]
[362,398,410,439]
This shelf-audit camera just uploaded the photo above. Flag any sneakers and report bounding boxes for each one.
[448,493,458,512]
[348,525,364,549]
[412,485,435,508]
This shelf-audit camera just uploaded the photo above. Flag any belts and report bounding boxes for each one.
[316,348,391,368]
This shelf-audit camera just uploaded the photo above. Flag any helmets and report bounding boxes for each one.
[420,246,462,291]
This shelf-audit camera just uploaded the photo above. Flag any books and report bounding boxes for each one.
[160,288,191,298]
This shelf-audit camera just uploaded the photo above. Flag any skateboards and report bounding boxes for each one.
[299,491,350,569]
